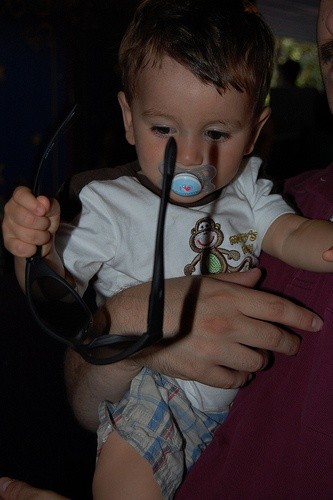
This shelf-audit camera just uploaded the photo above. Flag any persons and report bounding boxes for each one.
[65,0,333,500]
[3,3,333,500]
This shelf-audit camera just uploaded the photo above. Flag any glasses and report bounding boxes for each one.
[24,100,178,366]
